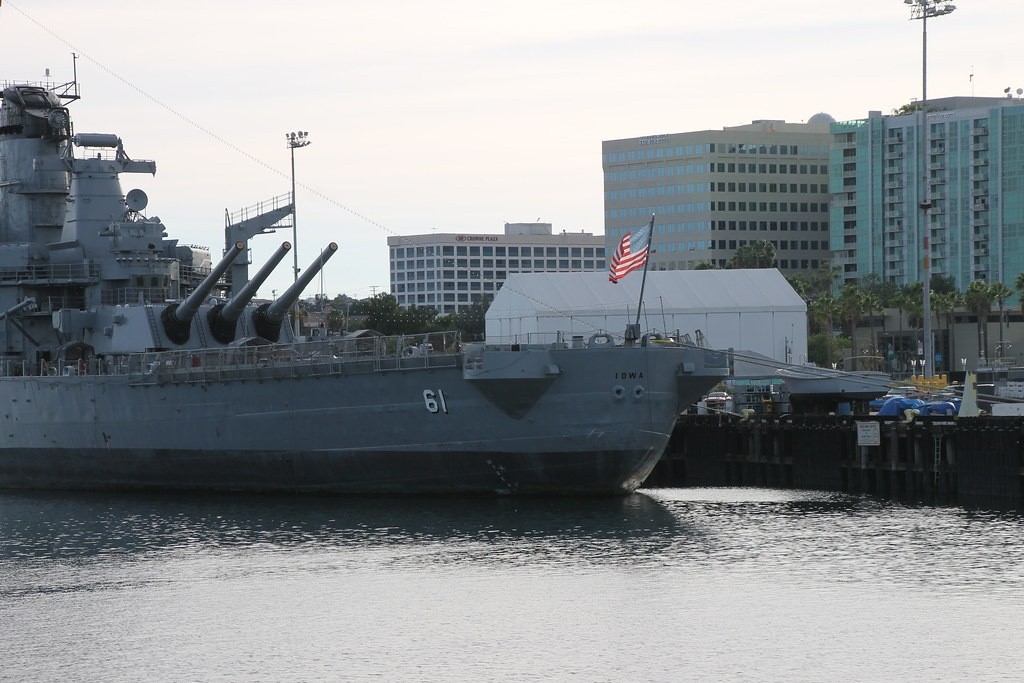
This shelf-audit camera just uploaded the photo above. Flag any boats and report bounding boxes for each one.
[868,385,963,417]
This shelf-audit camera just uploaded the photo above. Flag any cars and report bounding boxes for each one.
[704,391,732,407]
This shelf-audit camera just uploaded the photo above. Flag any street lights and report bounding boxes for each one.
[285,130,312,343]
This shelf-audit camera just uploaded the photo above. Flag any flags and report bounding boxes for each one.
[608,218,652,283]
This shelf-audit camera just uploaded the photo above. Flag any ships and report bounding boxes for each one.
[0,50,732,510]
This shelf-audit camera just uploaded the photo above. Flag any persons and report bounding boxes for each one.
[715,407,726,414]
[455,342,462,353]
